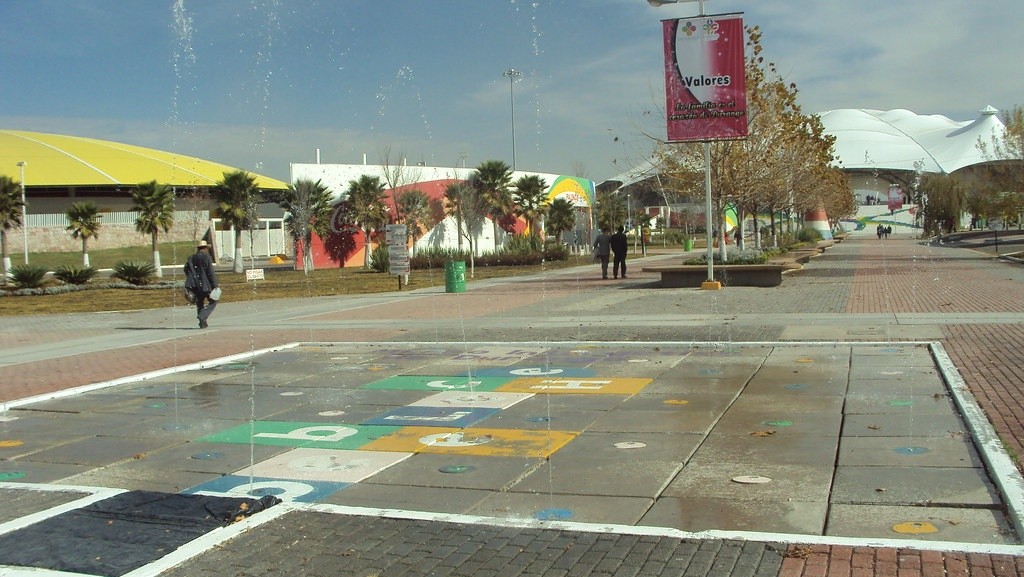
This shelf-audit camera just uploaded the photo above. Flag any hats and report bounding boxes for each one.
[192,240,211,249]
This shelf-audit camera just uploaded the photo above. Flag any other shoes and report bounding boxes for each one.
[602,276,629,280]
[197,316,207,328]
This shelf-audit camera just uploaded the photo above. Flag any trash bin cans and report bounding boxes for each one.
[683,239,691,252]
[444,260,466,293]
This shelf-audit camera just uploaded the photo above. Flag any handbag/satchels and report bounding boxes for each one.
[185,289,196,303]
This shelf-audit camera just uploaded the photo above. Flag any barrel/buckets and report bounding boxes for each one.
[684,238,692,252]
[445,261,467,293]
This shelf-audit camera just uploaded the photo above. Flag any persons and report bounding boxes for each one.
[903,196,906,203]
[184,240,220,328]
[734,228,742,247]
[610,226,628,280]
[877,223,892,239]
[866,194,880,205]
[593,224,612,280]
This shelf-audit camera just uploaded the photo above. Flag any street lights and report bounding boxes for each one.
[17,161,28,266]
[502,69,521,171]
[645,0,722,291]
